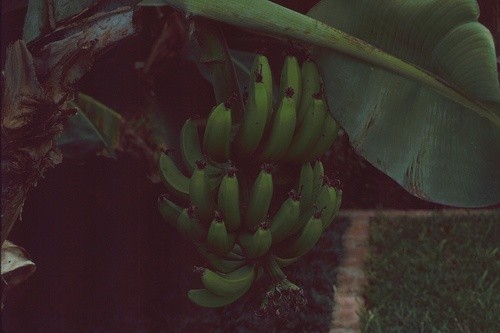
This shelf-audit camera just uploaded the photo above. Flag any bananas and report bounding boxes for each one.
[157,53,344,309]
